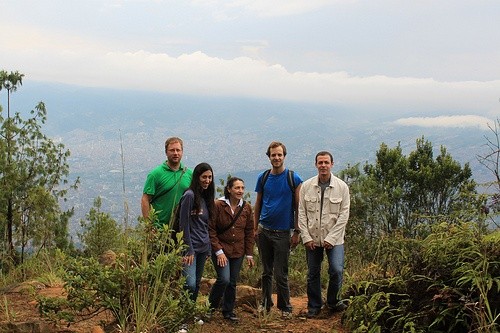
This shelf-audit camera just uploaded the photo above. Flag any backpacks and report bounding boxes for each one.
[165,201,181,251]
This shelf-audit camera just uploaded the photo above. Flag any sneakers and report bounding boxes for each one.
[222,309,240,321]
[178,328,188,333]
[193,319,204,326]
[203,307,215,321]
[305,307,322,319]
[281,311,293,319]
[326,302,348,312]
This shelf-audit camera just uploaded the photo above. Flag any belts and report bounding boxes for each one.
[258,223,290,234]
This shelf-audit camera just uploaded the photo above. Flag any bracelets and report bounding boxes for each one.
[294,230,301,234]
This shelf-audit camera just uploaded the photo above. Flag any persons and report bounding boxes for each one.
[206,177,254,323]
[298,151,350,318]
[254,141,304,319]
[141,137,194,317]
[178,162,215,333]
